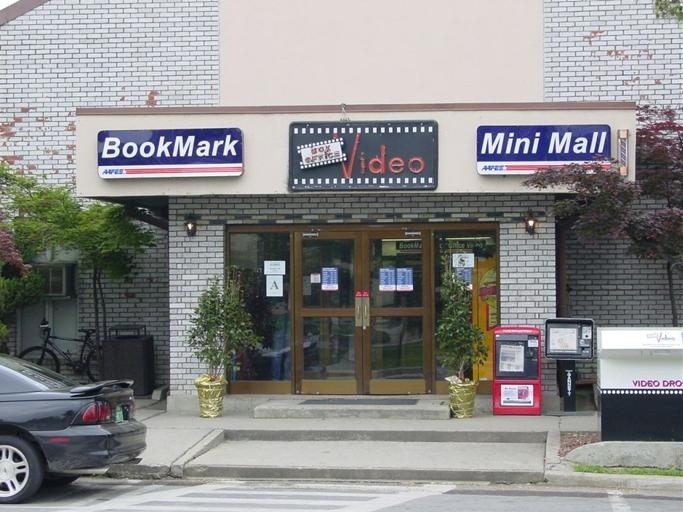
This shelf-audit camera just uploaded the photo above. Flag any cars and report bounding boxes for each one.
[246,316,423,380]
[0,352,146,504]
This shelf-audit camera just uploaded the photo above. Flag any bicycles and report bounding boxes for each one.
[19,326,103,382]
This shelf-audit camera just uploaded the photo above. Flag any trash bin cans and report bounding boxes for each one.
[102,324,154,396]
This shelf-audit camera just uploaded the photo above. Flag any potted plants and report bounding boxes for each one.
[435,250,488,416]
[187,264,263,416]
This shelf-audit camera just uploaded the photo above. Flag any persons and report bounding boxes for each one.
[265,281,292,382]
